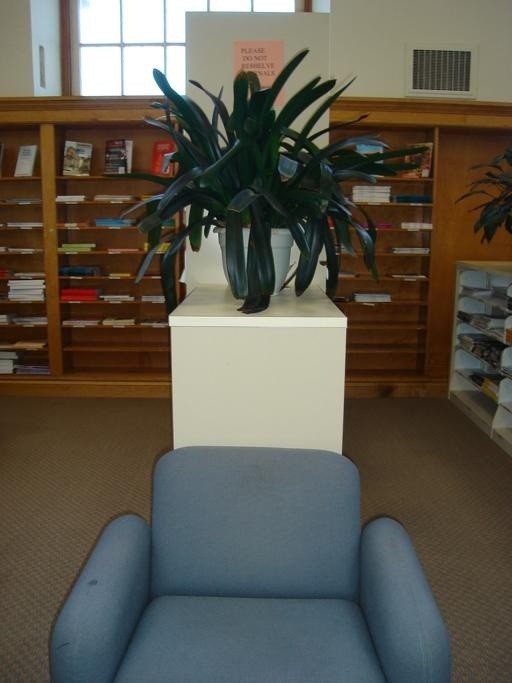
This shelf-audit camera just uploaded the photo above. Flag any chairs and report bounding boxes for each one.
[47,443,452,683]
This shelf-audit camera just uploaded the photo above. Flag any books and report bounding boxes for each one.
[457,285,512,402]
[329,142,434,302]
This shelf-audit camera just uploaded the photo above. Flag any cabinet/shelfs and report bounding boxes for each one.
[328,124,440,402]
[1,94,183,401]
[446,257,512,462]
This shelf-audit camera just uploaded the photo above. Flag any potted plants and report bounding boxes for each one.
[102,47,429,315]
